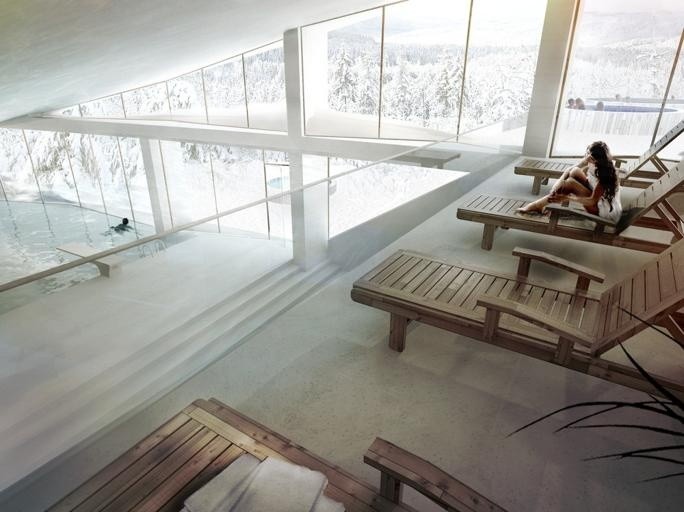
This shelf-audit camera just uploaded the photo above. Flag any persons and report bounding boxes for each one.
[513,139,621,227]
[100,217,137,237]
[567,93,632,111]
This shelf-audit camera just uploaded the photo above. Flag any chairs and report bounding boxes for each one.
[41,395,514,512]
[454,157,684,251]
[349,232,684,405]
[513,116,683,196]
